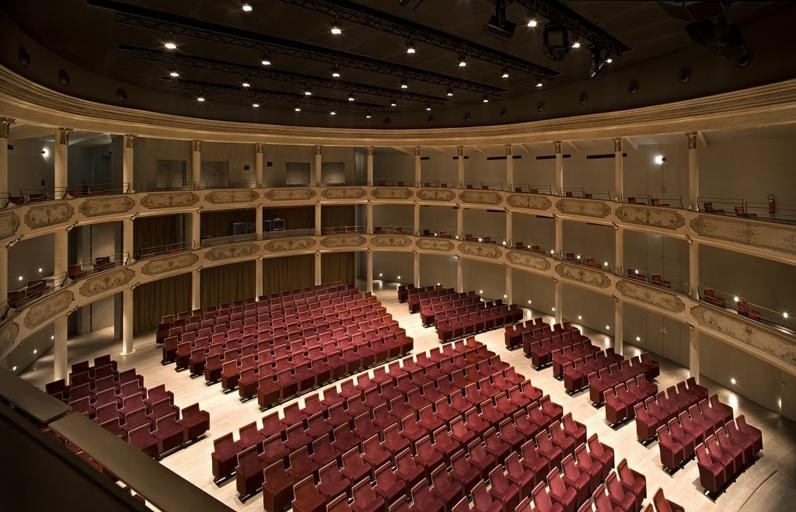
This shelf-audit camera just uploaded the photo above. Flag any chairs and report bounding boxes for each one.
[330,223,665,280]
[2,189,116,307]
[367,177,763,224]
[40,280,765,512]
[155,280,415,408]
[395,279,526,345]
[210,336,685,510]
[503,314,765,497]
[40,351,212,461]
